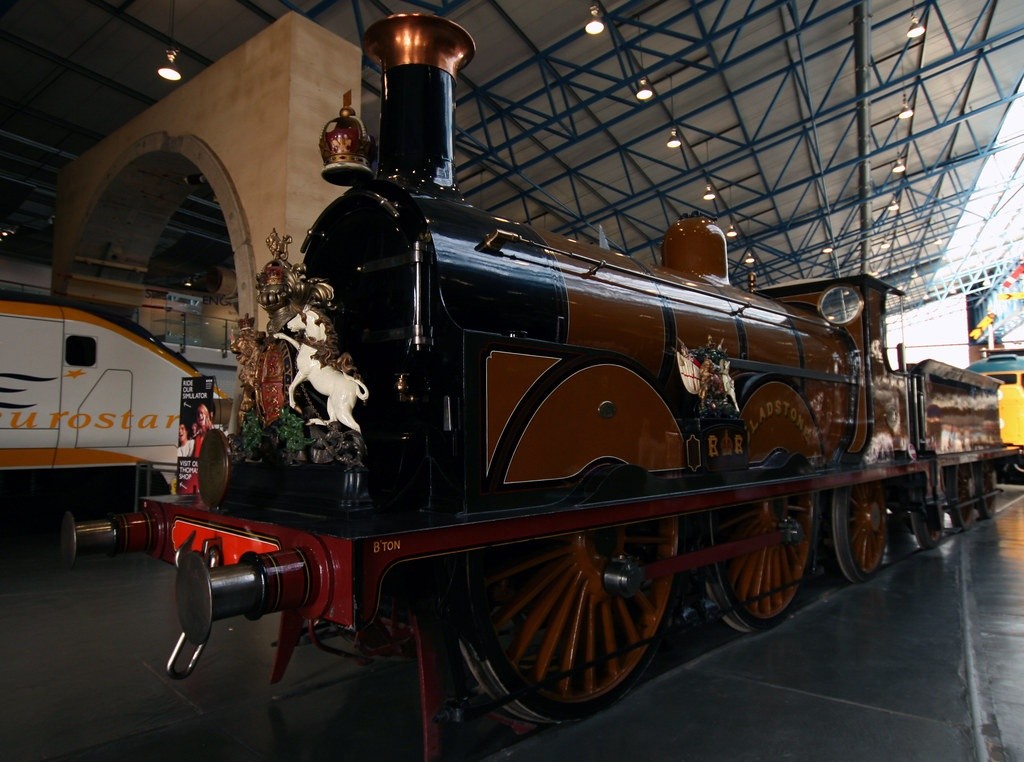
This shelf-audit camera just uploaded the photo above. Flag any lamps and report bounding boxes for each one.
[821,206,833,254]
[585,0,605,35]
[886,0,926,213]
[157,0,184,82]
[666,71,682,149]
[635,14,654,100]
[703,140,716,201]
[726,185,738,238]
[744,220,756,264]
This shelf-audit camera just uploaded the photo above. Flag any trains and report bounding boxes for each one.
[1,286,228,554]
[120,10,1024,762]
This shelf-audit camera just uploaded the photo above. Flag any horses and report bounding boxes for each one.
[722,361,740,412]
[273,304,369,434]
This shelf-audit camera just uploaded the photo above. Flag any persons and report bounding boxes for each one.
[178,404,214,494]
